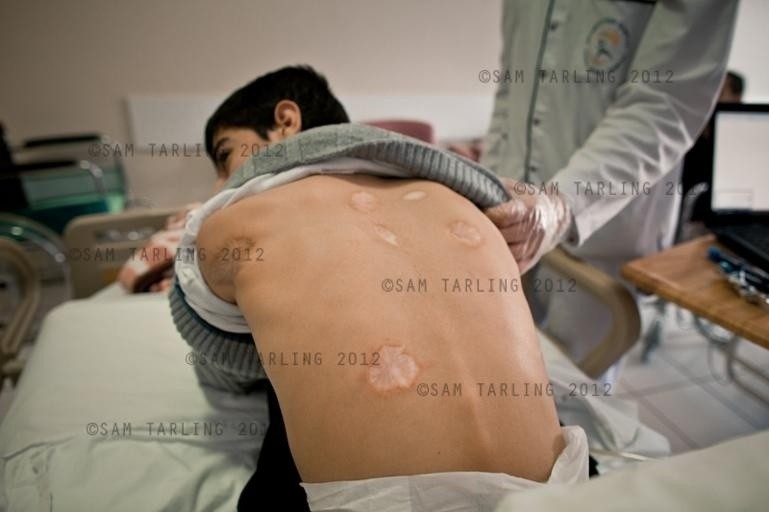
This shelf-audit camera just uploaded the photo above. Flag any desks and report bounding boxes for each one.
[619,232,769,409]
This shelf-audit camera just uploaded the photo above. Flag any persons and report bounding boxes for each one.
[719,72,744,104]
[477,0,741,278]
[165,63,591,512]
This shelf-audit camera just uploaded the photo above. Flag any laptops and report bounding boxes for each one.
[703,102,769,272]
